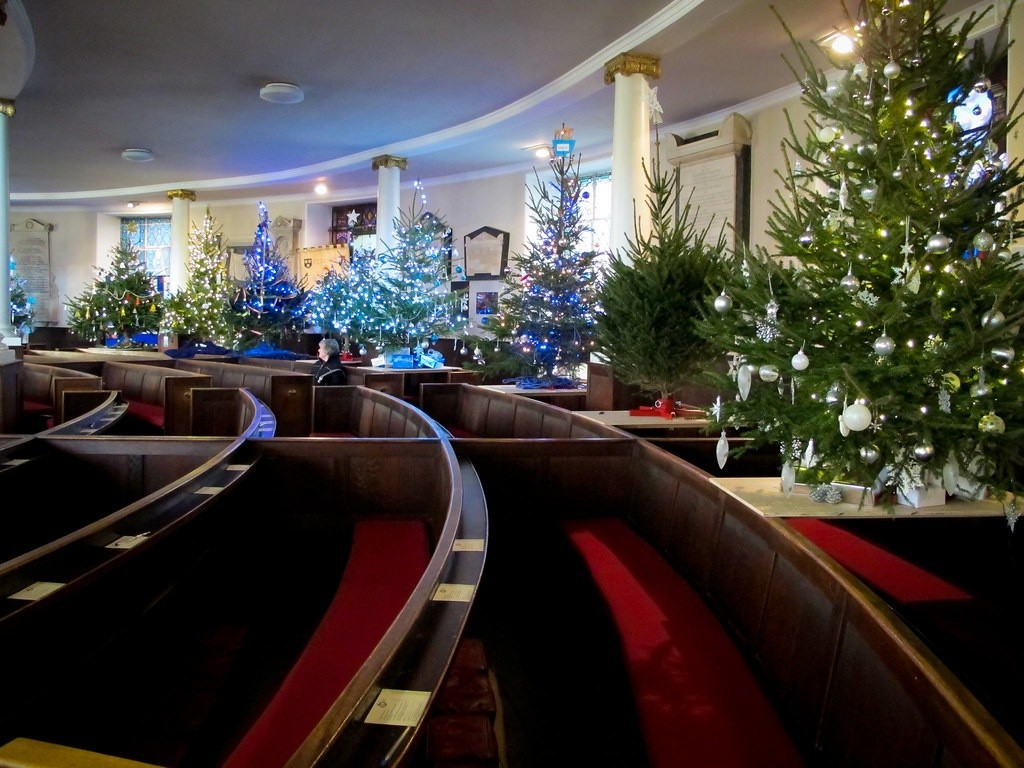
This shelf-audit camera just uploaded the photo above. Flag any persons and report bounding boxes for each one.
[306,338,346,386]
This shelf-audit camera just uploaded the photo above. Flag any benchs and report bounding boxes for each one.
[0,360,1024,767]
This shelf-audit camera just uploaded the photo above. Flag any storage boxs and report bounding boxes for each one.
[779,468,989,508]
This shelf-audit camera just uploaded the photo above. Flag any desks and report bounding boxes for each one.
[477,384,586,396]
[575,409,713,429]
[709,476,1024,519]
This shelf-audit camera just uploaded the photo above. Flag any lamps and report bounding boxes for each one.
[122,148,154,163]
[259,82,305,104]
